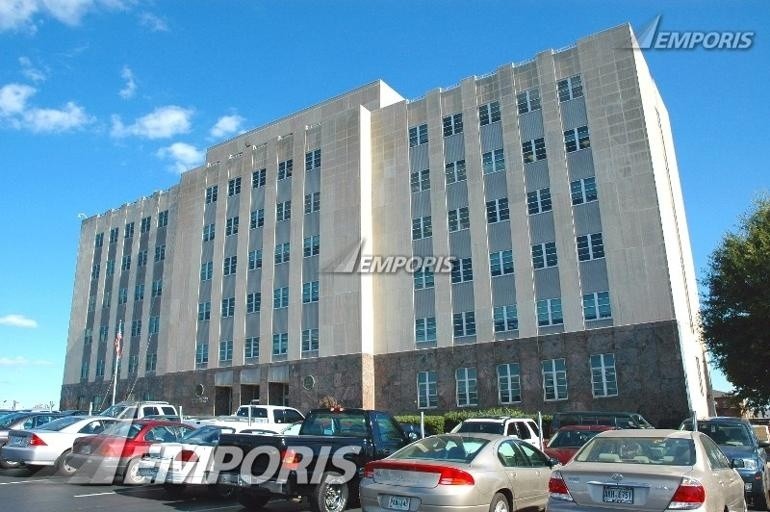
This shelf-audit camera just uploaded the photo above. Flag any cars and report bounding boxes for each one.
[0,399,770,512]
